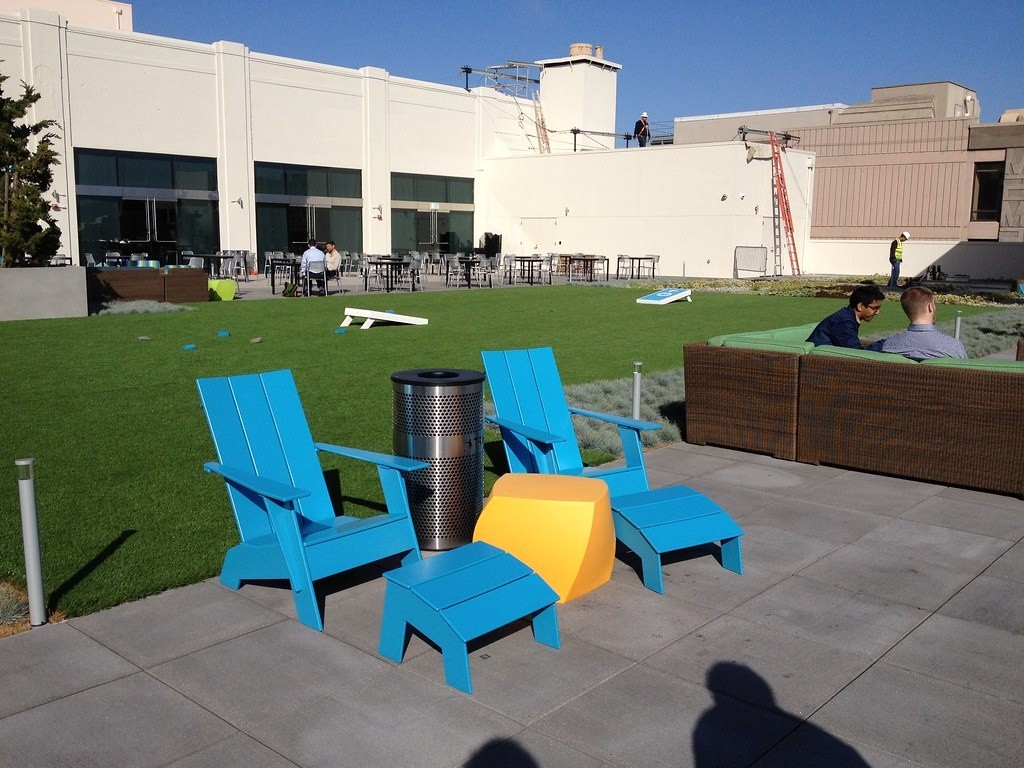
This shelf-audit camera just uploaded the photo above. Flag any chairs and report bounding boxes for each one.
[479,344,661,498]
[195,367,431,633]
[50,250,660,297]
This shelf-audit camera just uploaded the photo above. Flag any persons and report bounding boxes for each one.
[634,113,650,147]
[806,286,887,351]
[323,240,342,286]
[886,231,910,289]
[298,239,327,296]
[881,286,970,361]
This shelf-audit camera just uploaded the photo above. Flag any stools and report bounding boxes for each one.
[610,483,745,595]
[379,540,560,694]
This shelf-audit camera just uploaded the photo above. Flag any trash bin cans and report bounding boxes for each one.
[391,365,485,552]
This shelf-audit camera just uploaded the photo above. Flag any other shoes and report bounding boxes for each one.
[304,289,310,296]
[317,290,325,296]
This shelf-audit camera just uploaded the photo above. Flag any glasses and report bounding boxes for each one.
[863,303,880,311]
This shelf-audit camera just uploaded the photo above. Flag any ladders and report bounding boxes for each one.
[531,87,552,154]
[768,129,801,278]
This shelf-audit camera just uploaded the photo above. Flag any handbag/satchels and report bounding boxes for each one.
[283,282,298,296]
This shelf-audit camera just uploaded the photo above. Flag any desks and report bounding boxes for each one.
[568,257,609,282]
[445,260,493,289]
[616,257,655,280]
[182,254,234,276]
[508,259,551,286]
[364,262,416,293]
[271,262,304,295]
[104,255,132,267]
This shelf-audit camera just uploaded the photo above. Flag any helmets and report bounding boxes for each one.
[902,232,910,240]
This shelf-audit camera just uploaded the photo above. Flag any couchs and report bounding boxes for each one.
[683,321,1024,498]
